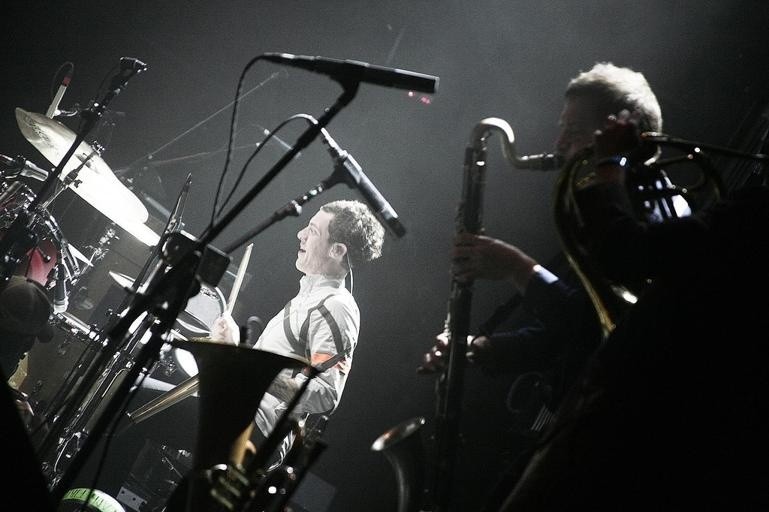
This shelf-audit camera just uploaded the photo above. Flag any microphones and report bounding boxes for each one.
[0,152,49,182]
[45,71,74,120]
[268,53,439,97]
[327,143,406,242]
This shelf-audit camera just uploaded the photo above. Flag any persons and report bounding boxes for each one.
[496,186,769,512]
[96,197,386,498]
[0,275,55,418]
[419,59,694,490]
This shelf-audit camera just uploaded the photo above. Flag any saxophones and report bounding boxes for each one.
[371,119,558,506]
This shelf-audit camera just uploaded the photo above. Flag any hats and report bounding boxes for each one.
[2,274,55,343]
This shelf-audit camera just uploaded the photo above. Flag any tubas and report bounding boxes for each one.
[551,134,768,339]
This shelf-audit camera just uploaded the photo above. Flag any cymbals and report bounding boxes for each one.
[14,101,132,198]
[53,171,176,245]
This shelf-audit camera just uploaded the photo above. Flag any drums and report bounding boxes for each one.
[2,182,80,296]
[149,348,198,383]
[161,265,227,338]
